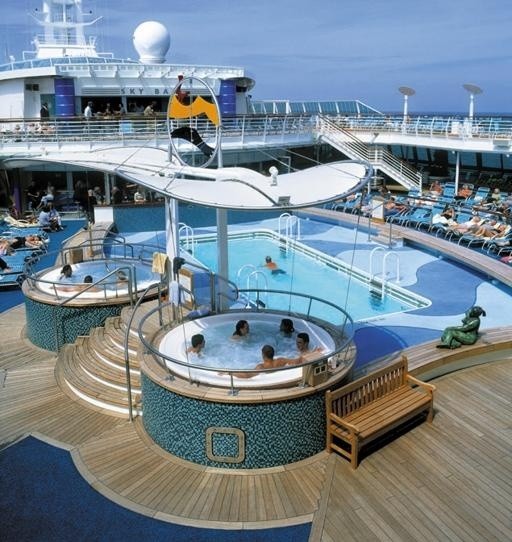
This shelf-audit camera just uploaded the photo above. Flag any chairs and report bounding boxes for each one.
[315,181,512,263]
[238,116,512,139]
[0,212,75,289]
[119,120,134,136]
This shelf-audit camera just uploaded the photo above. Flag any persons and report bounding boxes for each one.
[116,270,128,285]
[50,275,101,292]
[0,243,12,256]
[279,319,295,336]
[230,320,249,339]
[385,113,391,125]
[81,99,156,135]
[186,334,206,355]
[0,238,36,249]
[437,306,486,349]
[72,178,145,221]
[40,102,49,117]
[402,115,411,127]
[7,122,54,133]
[219,344,321,378]
[264,256,286,274]
[6,175,64,233]
[0,258,11,272]
[60,265,72,280]
[289,333,320,365]
[361,179,511,253]
[26,237,42,240]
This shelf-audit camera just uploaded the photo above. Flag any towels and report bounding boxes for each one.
[188,310,209,319]
[168,282,186,307]
[151,252,168,274]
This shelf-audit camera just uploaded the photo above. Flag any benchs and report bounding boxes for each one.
[325,356,437,470]
